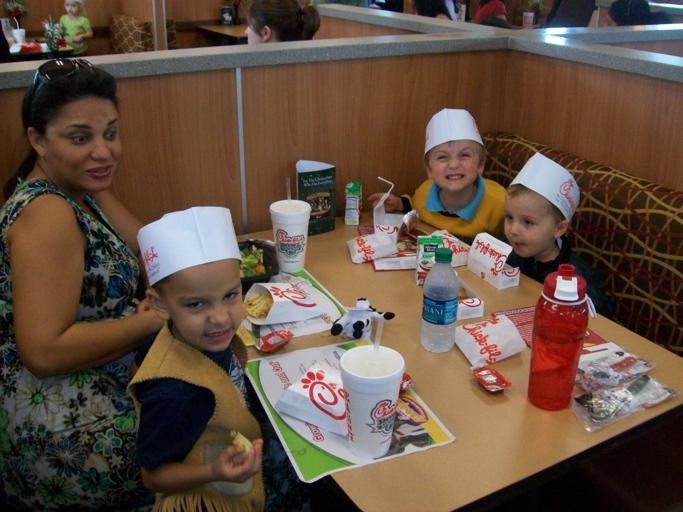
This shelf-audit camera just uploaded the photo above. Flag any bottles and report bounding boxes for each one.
[527,264,596,410]
[418,249,458,353]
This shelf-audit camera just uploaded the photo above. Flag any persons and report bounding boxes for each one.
[367,108,509,238]
[0,58,162,512]
[372,0,653,28]
[245,1,320,44]
[504,151,616,317]
[126,205,267,512]
[59,0,94,56]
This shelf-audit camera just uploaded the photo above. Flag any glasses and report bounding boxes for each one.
[29,57,93,98]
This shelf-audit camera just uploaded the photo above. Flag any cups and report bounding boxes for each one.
[11,28,26,46]
[269,200,311,275]
[523,12,534,26]
[340,345,404,460]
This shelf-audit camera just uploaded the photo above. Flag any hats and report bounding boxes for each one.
[424,108,485,158]
[507,152,581,222]
[135,205,246,288]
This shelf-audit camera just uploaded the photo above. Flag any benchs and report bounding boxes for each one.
[108,13,154,54]
[483,131,683,512]
